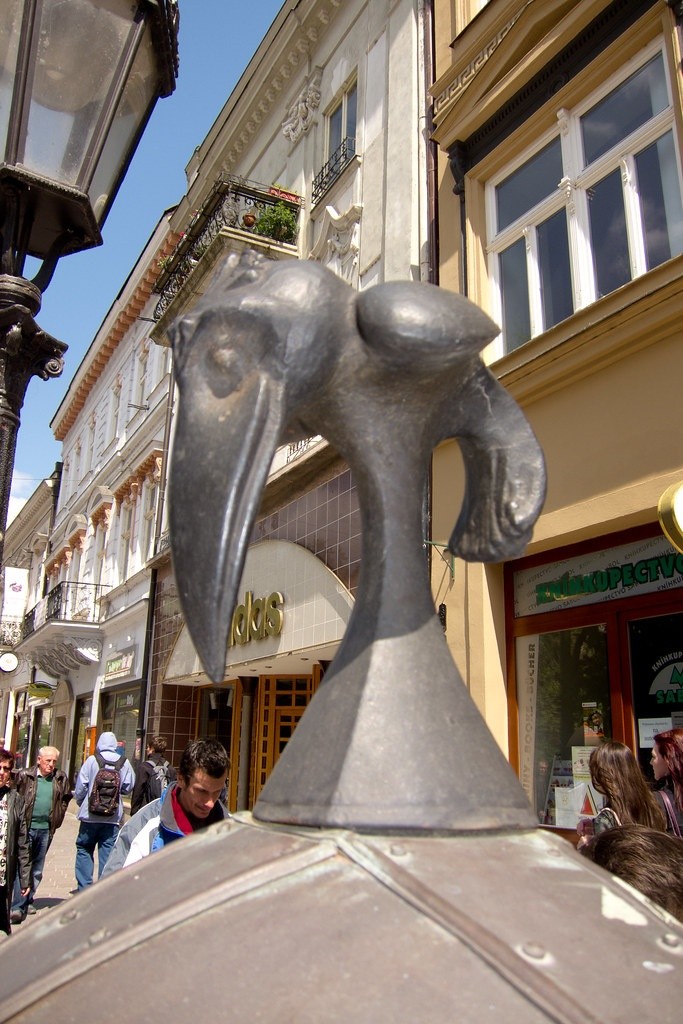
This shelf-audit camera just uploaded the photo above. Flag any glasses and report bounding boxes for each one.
[0,766,10,771]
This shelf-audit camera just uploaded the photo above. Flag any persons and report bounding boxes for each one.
[588,822,683,924]
[95,735,233,886]
[74,731,136,893]
[15,745,73,915]
[577,728,664,860]
[130,736,180,820]
[648,728,683,841]
[0,749,34,937]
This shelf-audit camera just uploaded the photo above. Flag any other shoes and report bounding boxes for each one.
[11,911,22,924]
[24,904,36,914]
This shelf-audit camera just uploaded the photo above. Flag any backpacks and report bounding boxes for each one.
[145,760,171,803]
[88,752,126,817]
